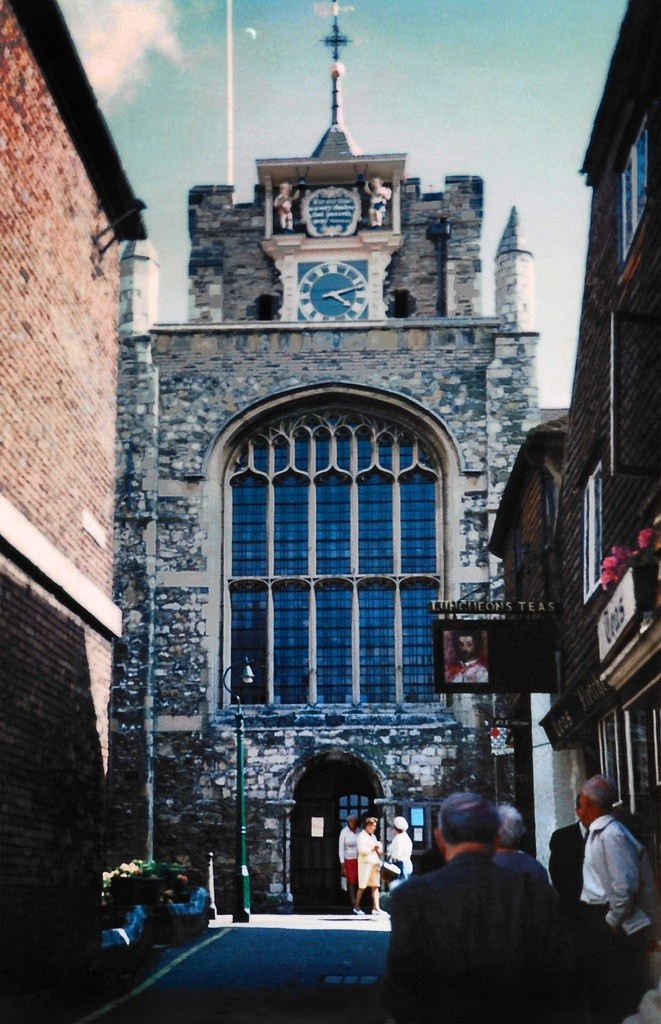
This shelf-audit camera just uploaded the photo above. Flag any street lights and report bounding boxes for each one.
[224,656,260,920]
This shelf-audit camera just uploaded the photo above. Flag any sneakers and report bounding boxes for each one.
[372,908,389,915]
[353,907,367,916]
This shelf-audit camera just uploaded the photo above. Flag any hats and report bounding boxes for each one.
[393,816,409,831]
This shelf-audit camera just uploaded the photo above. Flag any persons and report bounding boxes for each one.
[362,176,393,227]
[339,815,414,917]
[444,630,488,682]
[389,793,552,1024]
[547,776,655,1024]
[273,182,299,233]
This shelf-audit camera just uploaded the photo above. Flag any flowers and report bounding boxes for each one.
[599,529,654,584]
[100,854,198,926]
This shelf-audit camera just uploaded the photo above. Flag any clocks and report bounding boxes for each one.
[283,253,382,322]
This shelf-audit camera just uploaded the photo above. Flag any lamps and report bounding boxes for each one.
[639,610,659,633]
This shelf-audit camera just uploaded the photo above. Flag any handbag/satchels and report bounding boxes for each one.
[380,861,401,882]
[341,868,347,891]
